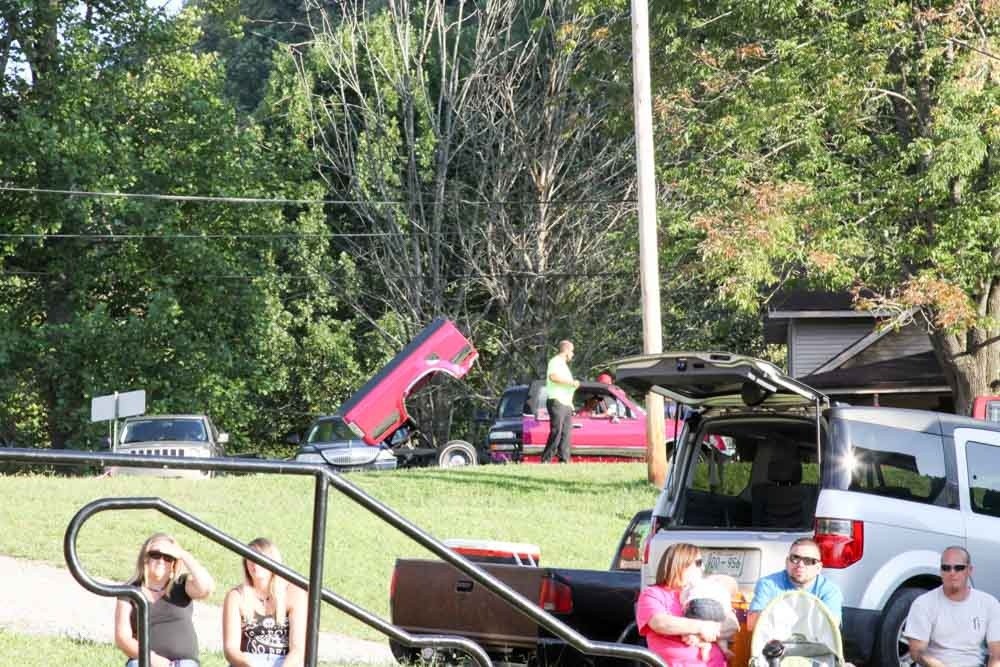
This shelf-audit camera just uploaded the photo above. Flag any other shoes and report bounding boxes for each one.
[560,461,568,464]
[542,460,551,464]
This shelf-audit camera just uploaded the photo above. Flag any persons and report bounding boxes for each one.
[583,372,626,418]
[540,340,580,464]
[115,532,216,667]
[747,538,843,632]
[990,380,1000,397]
[223,538,308,667]
[681,575,738,663]
[903,546,1000,667]
[636,543,741,667]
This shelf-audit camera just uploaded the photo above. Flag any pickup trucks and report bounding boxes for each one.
[388,509,664,667]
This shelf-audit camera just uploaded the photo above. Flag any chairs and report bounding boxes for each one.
[749,454,814,532]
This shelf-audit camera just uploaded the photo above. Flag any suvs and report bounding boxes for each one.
[587,351,1000,667]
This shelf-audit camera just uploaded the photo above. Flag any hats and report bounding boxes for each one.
[597,374,612,384]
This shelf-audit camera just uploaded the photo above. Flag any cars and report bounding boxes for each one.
[484,380,739,473]
[287,416,400,473]
[103,414,230,479]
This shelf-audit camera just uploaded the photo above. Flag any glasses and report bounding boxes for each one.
[146,551,174,562]
[695,558,702,568]
[941,563,968,571]
[789,554,820,566]
